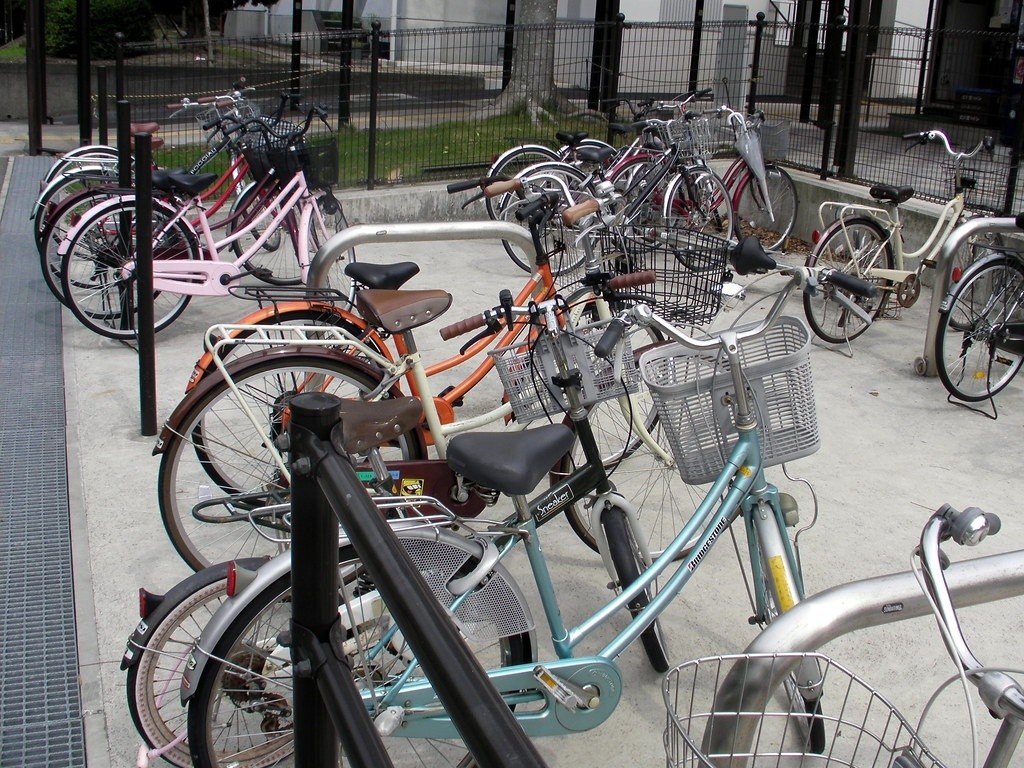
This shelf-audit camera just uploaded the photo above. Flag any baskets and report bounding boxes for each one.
[662,653,946,768]
[939,158,1018,213]
[639,316,820,485]
[487,318,639,424]
[195,97,261,151]
[542,224,730,329]
[242,134,339,192]
[636,110,718,159]
[746,117,791,162]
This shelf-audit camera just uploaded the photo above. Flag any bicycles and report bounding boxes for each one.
[30,77,1022,768]
